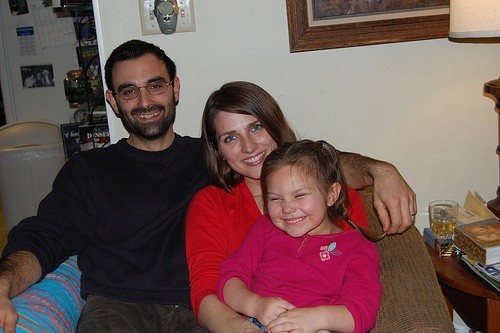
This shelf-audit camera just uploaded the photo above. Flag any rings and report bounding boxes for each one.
[411,214,416,216]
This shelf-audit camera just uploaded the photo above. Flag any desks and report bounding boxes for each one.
[421,236,500,333]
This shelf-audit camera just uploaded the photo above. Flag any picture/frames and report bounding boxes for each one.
[21,64,55,89]
[286,0,449,53]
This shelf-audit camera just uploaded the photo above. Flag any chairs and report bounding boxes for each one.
[0,183,456,333]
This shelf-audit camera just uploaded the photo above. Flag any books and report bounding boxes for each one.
[453,218,500,267]
[78,106,109,152]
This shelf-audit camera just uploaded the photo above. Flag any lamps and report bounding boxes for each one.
[449,0,500,218]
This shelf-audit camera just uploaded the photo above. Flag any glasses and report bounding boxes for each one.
[113,81,173,100]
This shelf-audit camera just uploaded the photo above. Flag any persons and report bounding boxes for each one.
[0,40,417,333]
[217,138,383,333]
[187,82,369,333]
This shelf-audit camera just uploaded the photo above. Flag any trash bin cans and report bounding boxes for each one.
[1,120,67,231]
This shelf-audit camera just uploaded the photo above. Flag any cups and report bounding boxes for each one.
[429,200,458,239]
